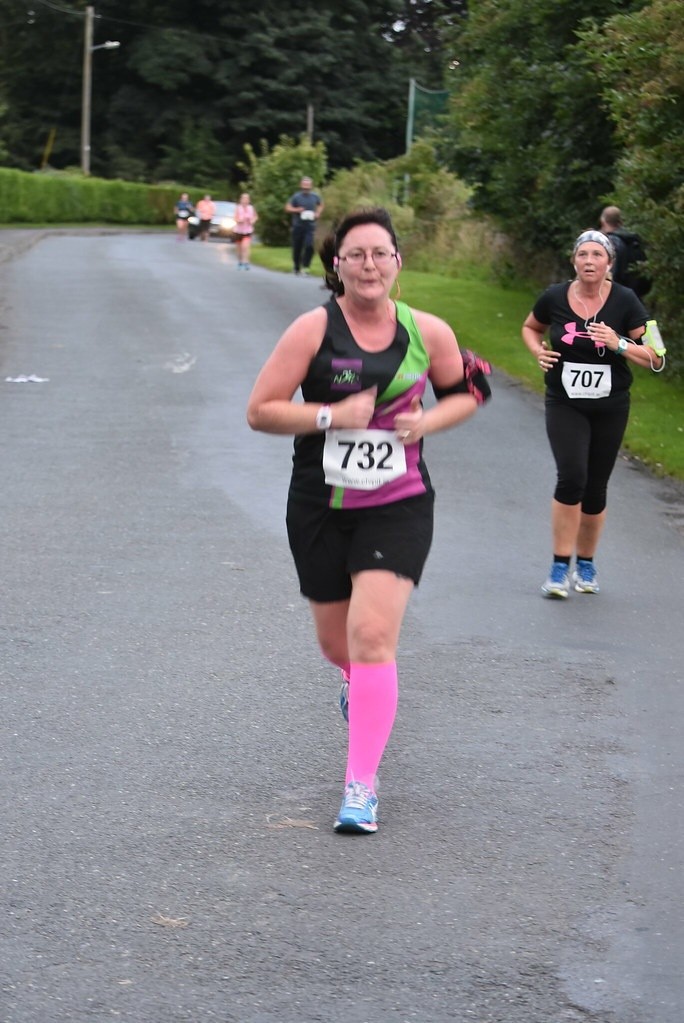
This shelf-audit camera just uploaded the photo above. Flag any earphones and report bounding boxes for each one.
[573,265,578,275]
[605,265,611,272]
[396,252,402,272]
[333,256,339,272]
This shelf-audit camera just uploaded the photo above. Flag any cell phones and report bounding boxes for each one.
[649,325,668,351]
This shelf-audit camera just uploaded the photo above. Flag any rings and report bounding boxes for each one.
[539,360,543,365]
[402,430,411,441]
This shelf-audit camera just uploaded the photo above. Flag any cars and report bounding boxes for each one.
[187,200,242,240]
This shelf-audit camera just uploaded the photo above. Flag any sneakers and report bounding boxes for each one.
[333,770,378,833]
[541,563,570,599]
[339,668,349,722]
[572,560,600,593]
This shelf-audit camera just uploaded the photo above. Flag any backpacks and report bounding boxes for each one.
[606,231,652,297]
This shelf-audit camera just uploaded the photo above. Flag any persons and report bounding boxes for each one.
[196,194,216,241]
[233,193,259,272]
[245,210,494,835]
[599,205,629,290]
[174,193,195,243]
[285,176,324,275]
[523,228,665,601]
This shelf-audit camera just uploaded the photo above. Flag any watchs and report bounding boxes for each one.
[614,337,628,355]
[315,401,334,432]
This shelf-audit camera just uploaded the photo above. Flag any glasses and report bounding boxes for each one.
[338,249,397,266]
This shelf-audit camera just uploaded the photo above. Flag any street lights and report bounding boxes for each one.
[81,39,122,177]
